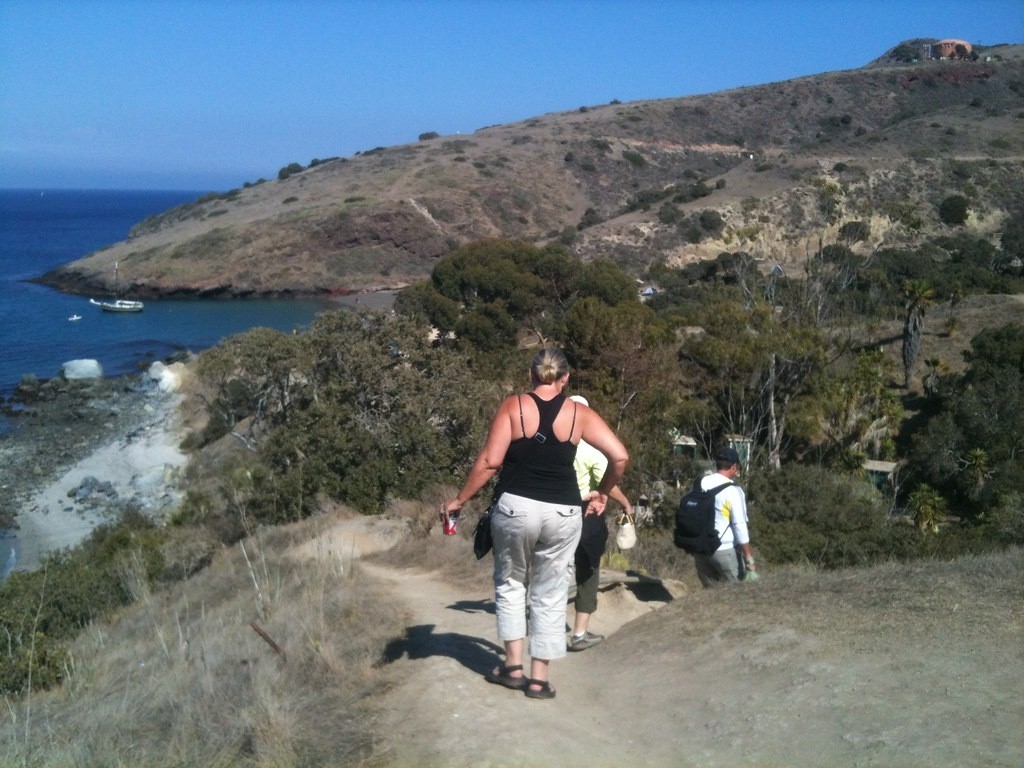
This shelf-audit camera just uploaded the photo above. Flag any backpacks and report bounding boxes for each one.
[673,477,740,556]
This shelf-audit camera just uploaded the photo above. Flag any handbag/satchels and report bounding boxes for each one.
[617,513,637,549]
[472,505,494,561]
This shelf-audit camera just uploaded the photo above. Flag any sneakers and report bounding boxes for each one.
[571,632,604,650]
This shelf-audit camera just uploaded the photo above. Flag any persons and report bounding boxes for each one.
[560,395,634,652]
[438,348,628,699]
[689,448,756,591]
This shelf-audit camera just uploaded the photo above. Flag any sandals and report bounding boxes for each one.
[525,679,556,699]
[486,664,525,687]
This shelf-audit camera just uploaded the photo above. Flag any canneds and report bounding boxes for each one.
[443,512,458,535]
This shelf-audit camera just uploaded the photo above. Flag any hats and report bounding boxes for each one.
[570,395,589,407]
[718,449,742,467]
